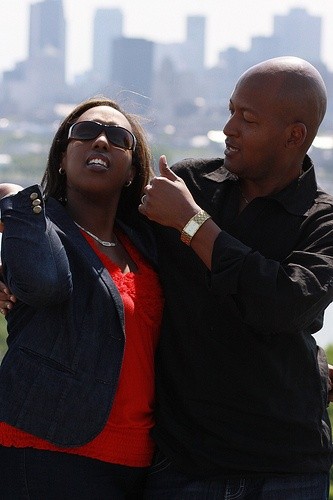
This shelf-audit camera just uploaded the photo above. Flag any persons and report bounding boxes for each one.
[0,55,333,500]
[0,97,333,500]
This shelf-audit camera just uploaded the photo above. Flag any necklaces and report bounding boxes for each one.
[73,220,121,247]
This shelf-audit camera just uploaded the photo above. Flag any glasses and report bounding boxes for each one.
[64,120,139,155]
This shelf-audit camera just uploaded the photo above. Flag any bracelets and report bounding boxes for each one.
[180,210,212,247]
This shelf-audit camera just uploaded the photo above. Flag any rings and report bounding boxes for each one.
[141,194,147,204]
